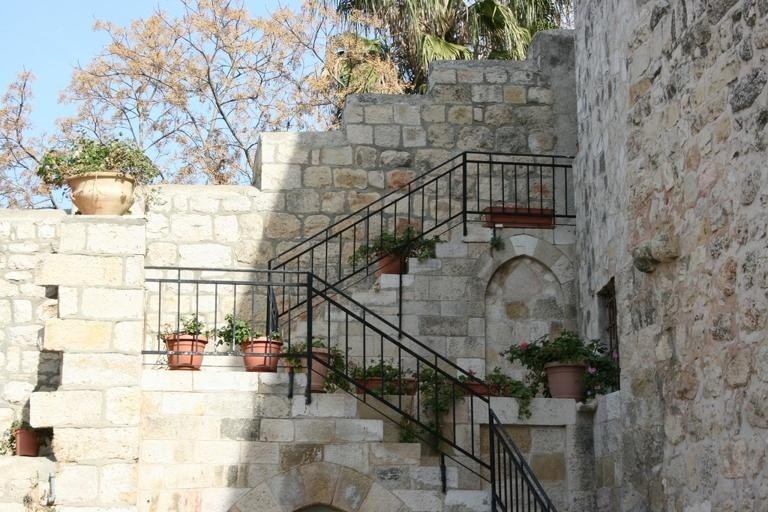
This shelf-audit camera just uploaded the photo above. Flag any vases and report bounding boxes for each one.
[545,360,590,400]
[479,204,557,230]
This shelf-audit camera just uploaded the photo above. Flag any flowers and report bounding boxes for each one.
[498,326,621,401]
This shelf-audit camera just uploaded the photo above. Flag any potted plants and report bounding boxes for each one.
[461,366,533,397]
[31,127,172,216]
[401,369,465,456]
[0,415,54,458]
[162,317,208,370]
[286,337,418,395]
[347,221,441,277]
[210,315,282,373]
